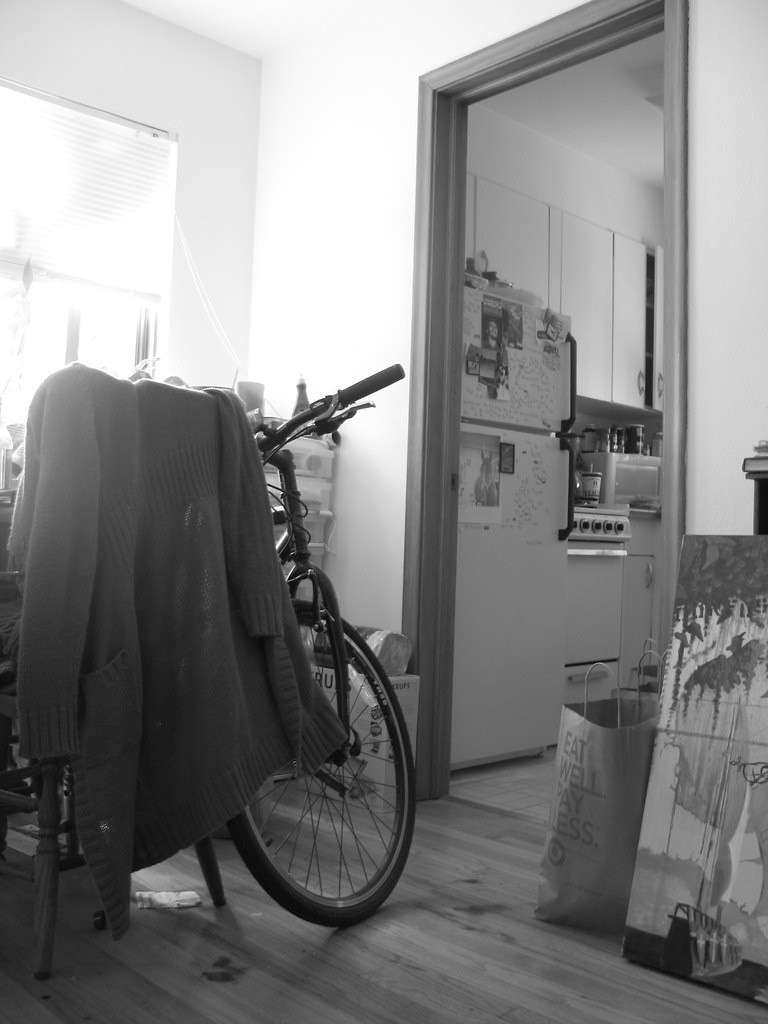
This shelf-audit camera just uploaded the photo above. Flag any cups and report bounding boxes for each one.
[581,473,602,504]
[236,382,264,414]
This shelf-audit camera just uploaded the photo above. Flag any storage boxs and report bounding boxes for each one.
[298,653,419,814]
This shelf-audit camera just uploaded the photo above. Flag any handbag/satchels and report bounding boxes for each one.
[532,649,663,938]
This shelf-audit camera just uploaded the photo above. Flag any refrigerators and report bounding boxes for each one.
[449,274,577,766]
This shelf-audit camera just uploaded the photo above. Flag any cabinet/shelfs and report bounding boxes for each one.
[262,450,337,602]
[465,172,665,422]
[564,513,664,708]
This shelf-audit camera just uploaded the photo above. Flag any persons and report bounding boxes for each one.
[482,321,499,349]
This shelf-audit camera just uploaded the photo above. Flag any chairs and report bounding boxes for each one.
[0,365,231,981]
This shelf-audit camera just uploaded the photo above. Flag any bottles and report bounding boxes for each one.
[292,383,313,432]
[651,432,663,457]
[581,424,598,453]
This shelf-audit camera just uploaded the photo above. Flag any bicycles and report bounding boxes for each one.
[225,363,418,928]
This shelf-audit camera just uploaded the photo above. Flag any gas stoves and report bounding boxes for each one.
[569,500,633,543]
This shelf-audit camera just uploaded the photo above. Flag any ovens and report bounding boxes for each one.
[566,544,627,704]
